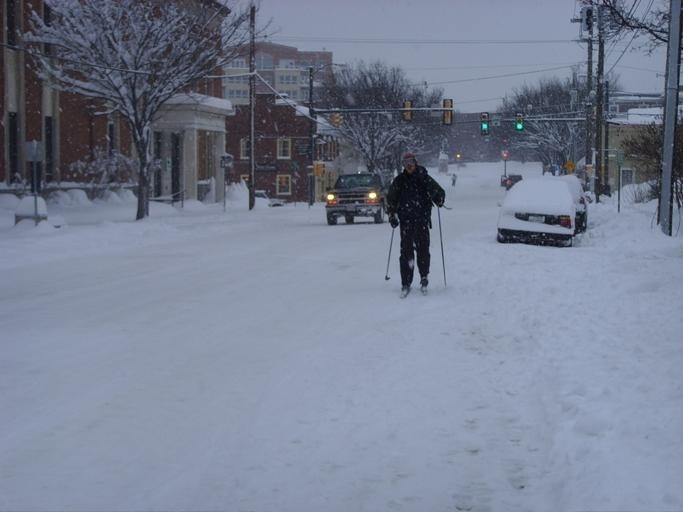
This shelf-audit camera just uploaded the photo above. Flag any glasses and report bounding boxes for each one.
[401,158,415,165]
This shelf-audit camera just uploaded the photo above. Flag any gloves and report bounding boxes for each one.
[390,218,397,227]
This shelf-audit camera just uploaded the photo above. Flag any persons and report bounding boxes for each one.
[451,173,457,187]
[386,152,445,296]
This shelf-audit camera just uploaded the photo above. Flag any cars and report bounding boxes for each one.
[496,173,586,248]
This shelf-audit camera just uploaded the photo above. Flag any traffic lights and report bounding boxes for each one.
[331,109,343,128]
[480,112,488,135]
[515,113,524,131]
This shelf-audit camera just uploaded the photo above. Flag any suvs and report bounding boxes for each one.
[325,171,387,225]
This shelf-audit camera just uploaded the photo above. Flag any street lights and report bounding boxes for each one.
[308,62,348,202]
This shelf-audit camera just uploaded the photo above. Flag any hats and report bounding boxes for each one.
[401,152,417,166]
